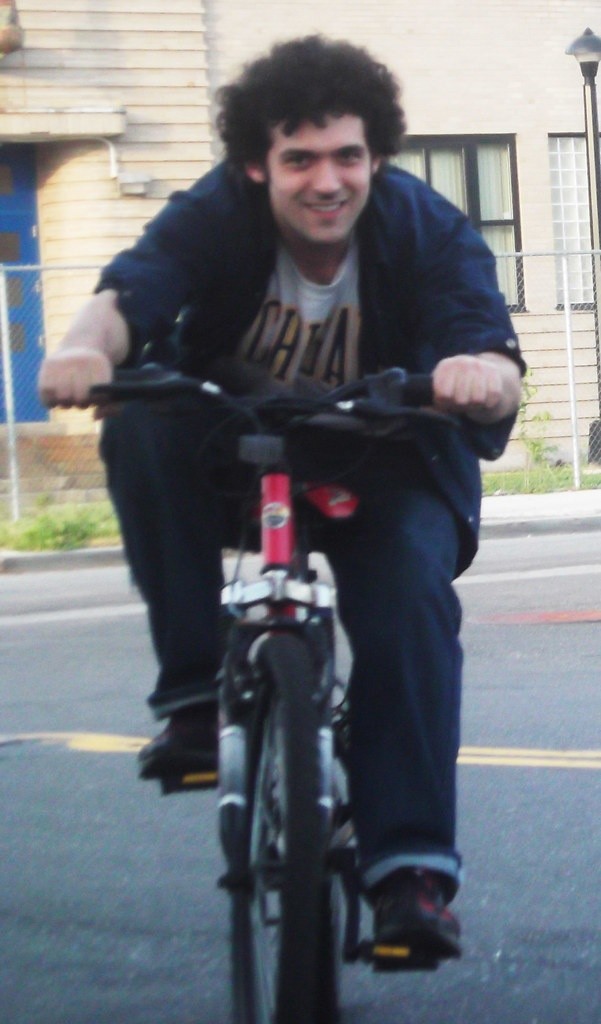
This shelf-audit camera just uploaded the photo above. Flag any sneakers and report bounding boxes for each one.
[373,866,462,940]
[137,710,220,779]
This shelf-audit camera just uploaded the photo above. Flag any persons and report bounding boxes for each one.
[37,34,527,962]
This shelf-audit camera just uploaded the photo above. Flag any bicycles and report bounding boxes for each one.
[85,366,443,1024]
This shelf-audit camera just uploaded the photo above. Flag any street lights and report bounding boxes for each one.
[568,27,601,475]
